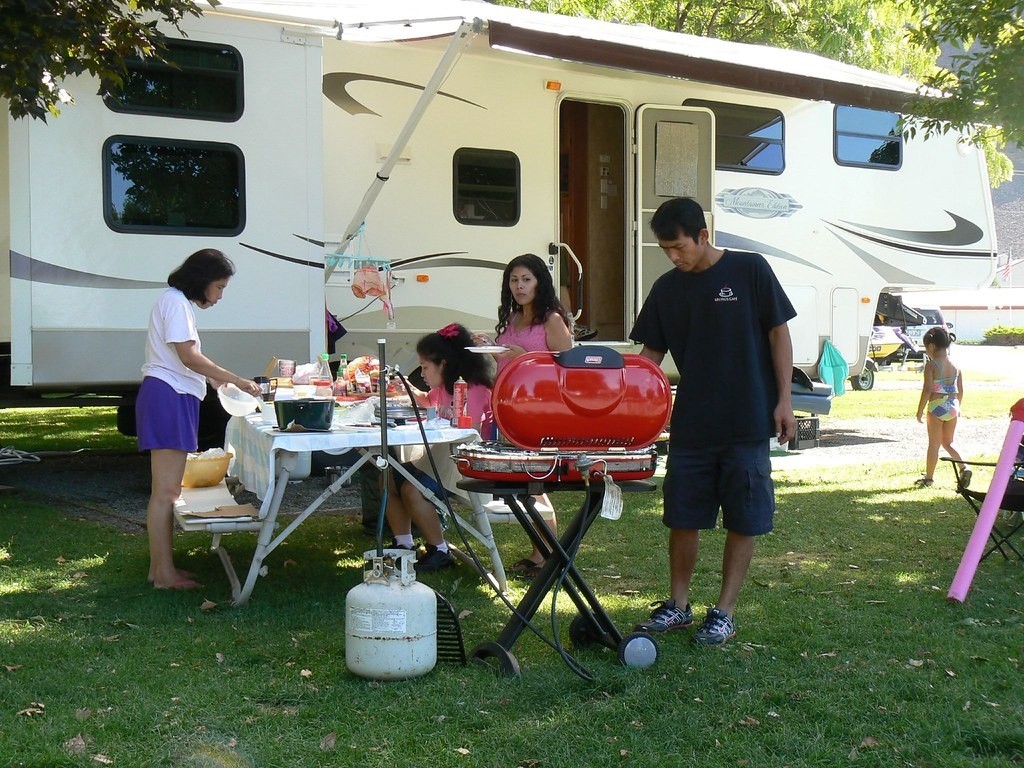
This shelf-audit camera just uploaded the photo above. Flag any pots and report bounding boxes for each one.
[274,400,335,430]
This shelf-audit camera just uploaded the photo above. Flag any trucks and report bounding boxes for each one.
[1,0,1001,478]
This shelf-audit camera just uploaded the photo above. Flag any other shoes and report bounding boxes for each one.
[916,472,934,487]
[954,467,972,493]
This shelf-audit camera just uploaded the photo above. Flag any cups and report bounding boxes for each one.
[270,379,277,400]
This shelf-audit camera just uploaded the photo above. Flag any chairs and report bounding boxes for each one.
[941,457,1024,562]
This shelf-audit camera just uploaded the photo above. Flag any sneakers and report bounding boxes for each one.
[387,537,427,565]
[689,608,735,647]
[414,543,457,570]
[633,598,693,633]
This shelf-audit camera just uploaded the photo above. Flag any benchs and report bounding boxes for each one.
[176,479,280,532]
[450,494,556,524]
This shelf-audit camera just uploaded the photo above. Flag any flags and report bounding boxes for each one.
[1003,252,1011,276]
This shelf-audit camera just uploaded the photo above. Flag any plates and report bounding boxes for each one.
[464,346,510,354]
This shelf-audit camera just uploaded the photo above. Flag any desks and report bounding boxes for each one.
[454,479,662,683]
[216,409,510,607]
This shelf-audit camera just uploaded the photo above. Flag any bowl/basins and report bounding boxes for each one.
[219,382,259,417]
[293,385,317,397]
[182,452,233,488]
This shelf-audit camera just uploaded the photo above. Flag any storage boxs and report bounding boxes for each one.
[789,416,820,449]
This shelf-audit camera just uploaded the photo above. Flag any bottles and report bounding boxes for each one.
[314,381,333,397]
[318,353,333,383]
[450,402,453,426]
[338,353,348,373]
[453,376,468,427]
[334,371,346,396]
[253,377,272,403]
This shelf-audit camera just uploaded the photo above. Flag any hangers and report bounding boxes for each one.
[326,222,391,271]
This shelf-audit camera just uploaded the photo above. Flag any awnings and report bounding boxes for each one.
[319,2,1013,288]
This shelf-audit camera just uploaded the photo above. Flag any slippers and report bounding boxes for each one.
[147,570,195,582]
[517,565,543,579]
[154,578,201,592]
[505,558,534,572]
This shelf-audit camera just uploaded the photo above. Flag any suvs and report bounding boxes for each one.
[904,303,953,359]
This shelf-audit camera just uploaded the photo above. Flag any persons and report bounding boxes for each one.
[559,250,598,341]
[380,254,573,576]
[917,327,972,493]
[135,248,261,590]
[631,198,797,647]
